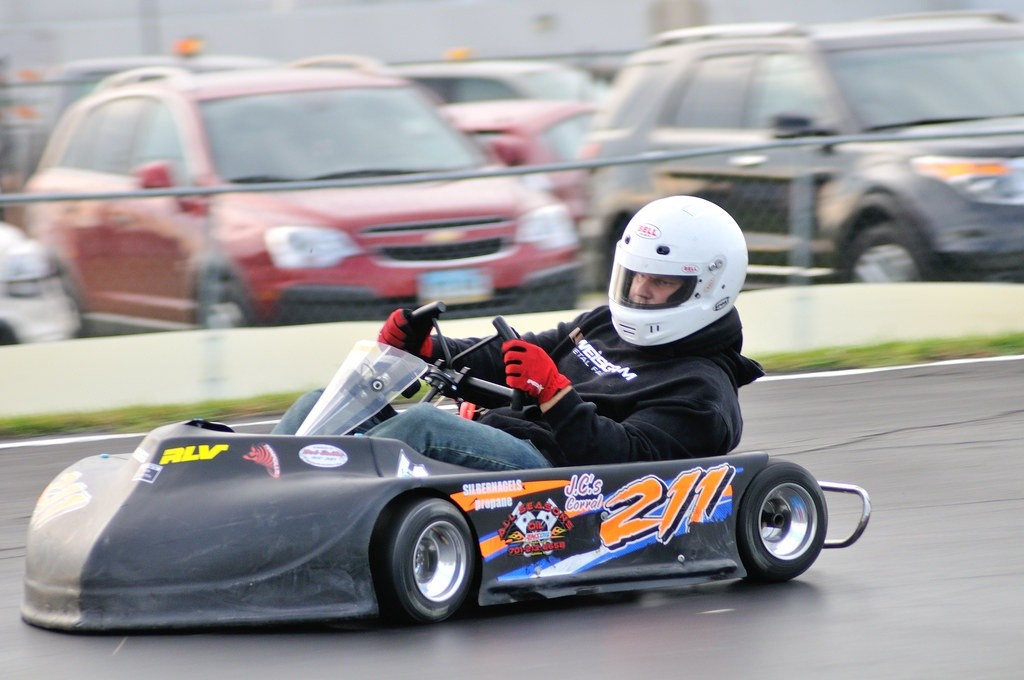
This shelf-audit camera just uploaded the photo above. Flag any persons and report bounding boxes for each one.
[270,196,765,471]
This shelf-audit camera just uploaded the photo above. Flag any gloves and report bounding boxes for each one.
[502,341,572,404]
[378,308,433,358]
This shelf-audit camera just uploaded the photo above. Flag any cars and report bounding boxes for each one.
[436,95,605,221]
[1,219,79,345]
[378,62,612,104]
[29,60,276,175]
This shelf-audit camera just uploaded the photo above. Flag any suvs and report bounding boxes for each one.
[16,60,582,339]
[577,11,1024,286]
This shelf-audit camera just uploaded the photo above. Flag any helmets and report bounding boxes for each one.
[609,194,748,346]
